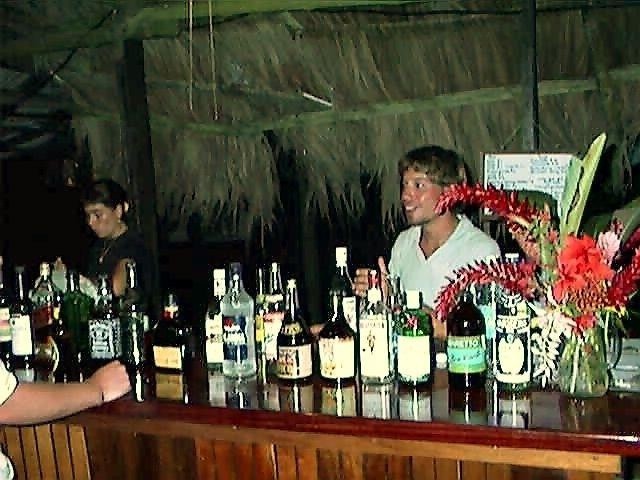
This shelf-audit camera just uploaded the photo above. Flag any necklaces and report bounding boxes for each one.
[99,238,114,265]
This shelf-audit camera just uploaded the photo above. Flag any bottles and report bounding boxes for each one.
[494,253,532,385]
[221,262,257,378]
[89,271,124,359]
[262,260,287,377]
[275,277,314,380]
[9,267,35,369]
[120,261,149,367]
[447,292,488,389]
[150,292,192,370]
[253,260,267,377]
[398,291,434,388]
[328,247,355,293]
[318,293,356,379]
[35,263,62,347]
[359,271,395,384]
[205,267,227,371]
[61,270,95,358]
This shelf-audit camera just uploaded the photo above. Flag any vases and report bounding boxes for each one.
[557,319,607,392]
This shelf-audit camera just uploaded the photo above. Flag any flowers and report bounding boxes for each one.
[428,131,638,392]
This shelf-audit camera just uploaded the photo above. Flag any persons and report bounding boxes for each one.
[81,178,155,303]
[0,358,132,480]
[352,145,503,342]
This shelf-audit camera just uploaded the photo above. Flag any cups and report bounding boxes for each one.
[33,343,53,383]
